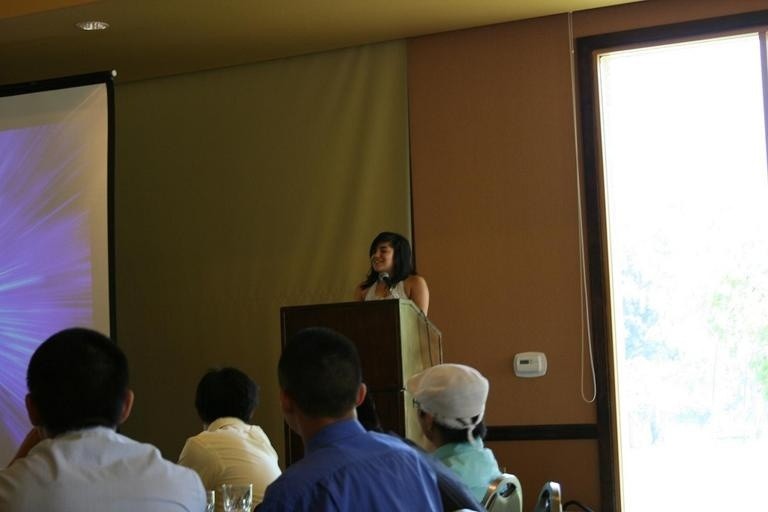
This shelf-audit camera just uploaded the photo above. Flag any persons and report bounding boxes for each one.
[354,231,428,316]
[1,327,207,512]
[407,361,502,505]
[254,325,489,512]
[177,366,283,512]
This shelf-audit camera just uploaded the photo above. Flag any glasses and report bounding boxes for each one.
[411,398,419,409]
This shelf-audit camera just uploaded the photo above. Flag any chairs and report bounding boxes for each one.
[532,479,564,512]
[479,472,527,511]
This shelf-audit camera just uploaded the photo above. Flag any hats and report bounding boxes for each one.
[407,363,490,431]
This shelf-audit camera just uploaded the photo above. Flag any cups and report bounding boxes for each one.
[222,480,252,511]
[204,489,215,512]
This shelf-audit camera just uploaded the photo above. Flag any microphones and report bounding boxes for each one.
[377,270,399,298]
